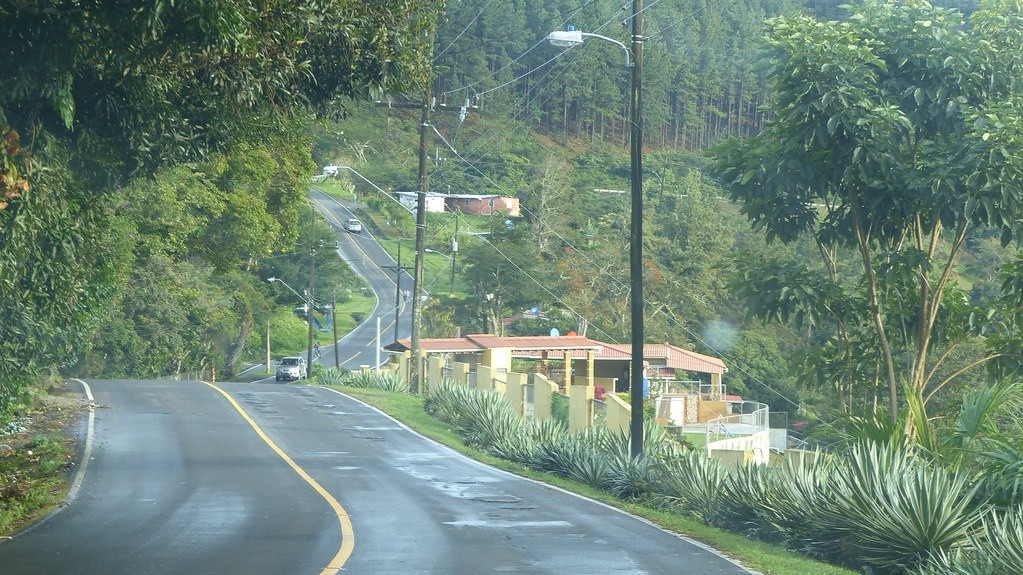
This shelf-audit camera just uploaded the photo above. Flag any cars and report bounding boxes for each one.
[344,218,362,234]
[276,357,308,381]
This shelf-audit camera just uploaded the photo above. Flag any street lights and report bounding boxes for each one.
[323,165,425,395]
[377,296,427,373]
[267,277,314,380]
[547,28,645,461]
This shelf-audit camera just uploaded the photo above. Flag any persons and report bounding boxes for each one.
[314,342,321,355]
[618,367,629,393]
